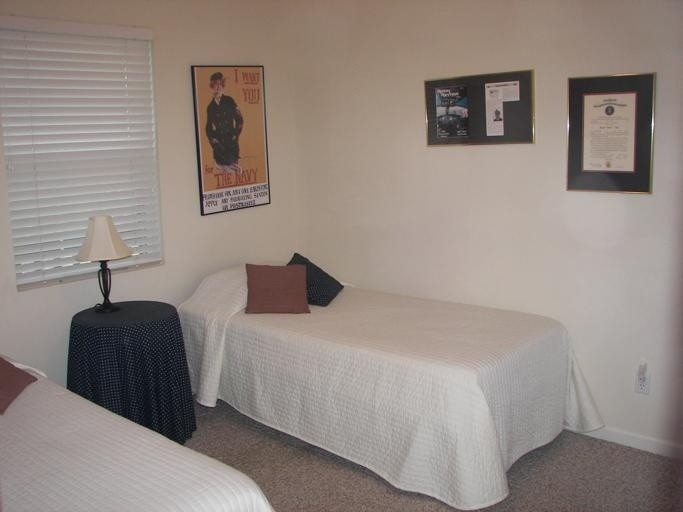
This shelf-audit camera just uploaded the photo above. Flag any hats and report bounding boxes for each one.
[210,72,222,81]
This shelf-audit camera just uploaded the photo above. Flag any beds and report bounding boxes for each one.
[0,354,274,511]
[176,264,606,510]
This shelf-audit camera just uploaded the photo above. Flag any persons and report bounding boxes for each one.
[494,109,502,121]
[205,72,243,171]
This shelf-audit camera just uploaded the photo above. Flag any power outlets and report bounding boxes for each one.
[634,367,651,395]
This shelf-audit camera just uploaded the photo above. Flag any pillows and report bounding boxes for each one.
[246,264,311,314]
[0,354,37,415]
[285,253,344,306]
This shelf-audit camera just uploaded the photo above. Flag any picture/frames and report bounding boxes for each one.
[191,65,270,217]
[567,73,656,195]
[424,69,535,146]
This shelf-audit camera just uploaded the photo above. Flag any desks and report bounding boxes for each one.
[67,301,198,445]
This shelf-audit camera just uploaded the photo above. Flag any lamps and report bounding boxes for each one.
[74,215,133,313]
